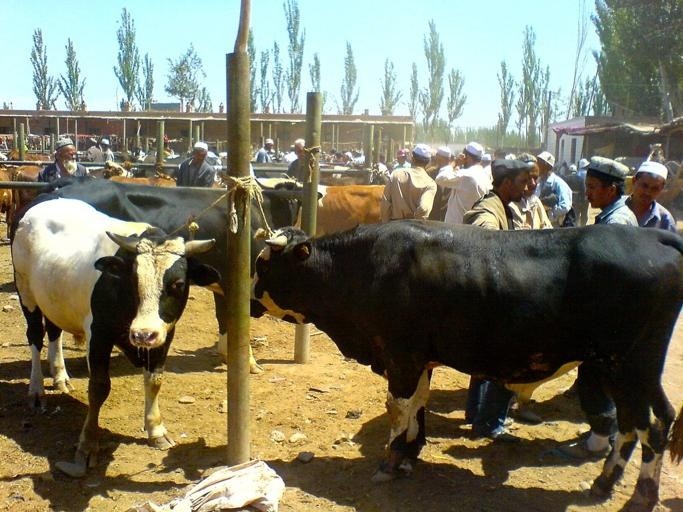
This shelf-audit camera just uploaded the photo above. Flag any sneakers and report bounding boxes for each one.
[486,431,520,443]
[557,437,613,459]
[505,418,514,426]
[554,385,580,411]
[517,409,542,424]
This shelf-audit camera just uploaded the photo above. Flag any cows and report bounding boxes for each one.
[108,175,229,189]
[0,150,54,240]
[249,217,683,512]
[49,179,323,374]
[316,184,386,238]
[13,160,136,218]
[12,197,221,469]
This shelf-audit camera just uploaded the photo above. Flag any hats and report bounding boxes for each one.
[194,142,209,151]
[537,151,668,180]
[397,142,492,161]
[54,137,109,150]
[266,139,274,144]
[492,160,529,184]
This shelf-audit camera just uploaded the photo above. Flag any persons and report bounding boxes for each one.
[34,139,88,187]
[85,138,118,164]
[372,142,677,460]
[136,138,307,188]
[318,148,366,176]
